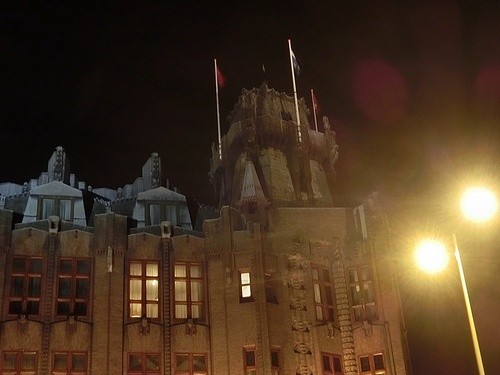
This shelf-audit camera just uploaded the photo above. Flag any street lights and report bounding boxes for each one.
[412,186,500,375]
